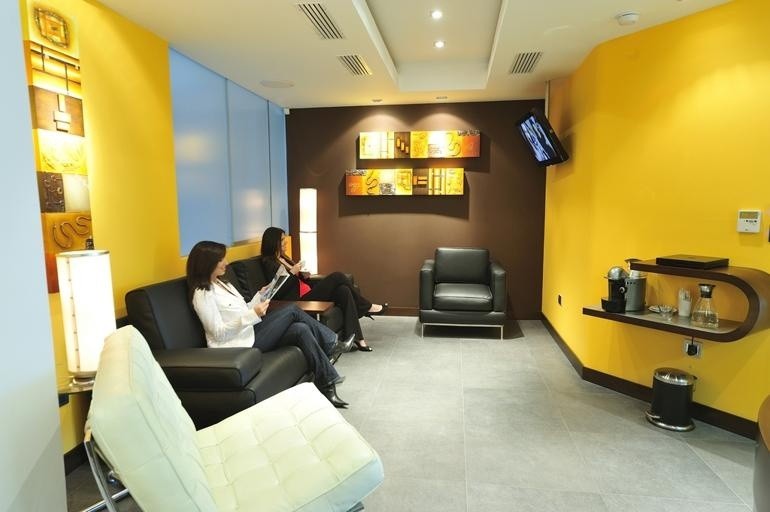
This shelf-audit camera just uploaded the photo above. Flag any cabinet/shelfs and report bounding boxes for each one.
[582,259,770,342]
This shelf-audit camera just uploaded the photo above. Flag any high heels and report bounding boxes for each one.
[354,339,372,351]
[330,333,356,361]
[365,302,389,320]
[320,383,349,406]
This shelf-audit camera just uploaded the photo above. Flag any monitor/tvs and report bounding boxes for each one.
[515,106,569,167]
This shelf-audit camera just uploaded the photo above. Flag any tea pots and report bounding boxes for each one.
[690,283,721,330]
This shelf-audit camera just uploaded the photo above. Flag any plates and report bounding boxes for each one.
[647,304,677,313]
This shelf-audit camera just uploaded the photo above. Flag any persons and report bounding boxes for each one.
[185,240,356,407]
[260,226,389,352]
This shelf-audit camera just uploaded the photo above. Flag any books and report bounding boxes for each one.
[263,263,290,300]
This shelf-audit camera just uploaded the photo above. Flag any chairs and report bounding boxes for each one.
[419,245,507,340]
[75,321,386,512]
[116,264,313,431]
[228,255,361,341]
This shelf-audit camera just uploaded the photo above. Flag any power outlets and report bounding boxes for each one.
[683,339,703,358]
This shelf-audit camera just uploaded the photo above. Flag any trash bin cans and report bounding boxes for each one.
[645,367,698,432]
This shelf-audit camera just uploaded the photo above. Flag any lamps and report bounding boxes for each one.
[55,250,118,386]
[298,186,318,276]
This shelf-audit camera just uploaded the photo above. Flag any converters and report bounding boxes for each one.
[687,344,698,357]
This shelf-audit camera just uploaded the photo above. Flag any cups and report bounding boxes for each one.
[658,304,674,320]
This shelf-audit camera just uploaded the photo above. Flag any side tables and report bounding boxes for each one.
[266,300,335,322]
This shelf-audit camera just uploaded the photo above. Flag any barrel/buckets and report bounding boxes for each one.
[603,276,647,312]
